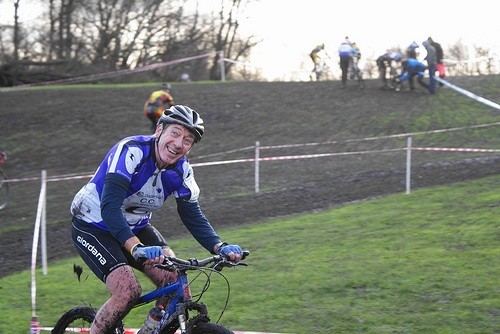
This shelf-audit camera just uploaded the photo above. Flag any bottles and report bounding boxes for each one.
[138,305,164,334]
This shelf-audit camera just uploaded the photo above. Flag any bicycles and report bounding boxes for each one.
[51,250,250,334]
[341,60,367,89]
[385,63,404,93]
[310,56,331,81]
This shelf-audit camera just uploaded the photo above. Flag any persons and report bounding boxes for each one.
[143,83,175,133]
[338,35,361,88]
[395,58,431,92]
[427,37,446,86]
[69,105,244,334]
[376,50,403,90]
[309,43,325,83]
[422,41,440,94]
[404,40,419,60]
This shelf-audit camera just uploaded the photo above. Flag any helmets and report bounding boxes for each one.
[160,84,171,90]
[156,105,204,143]
